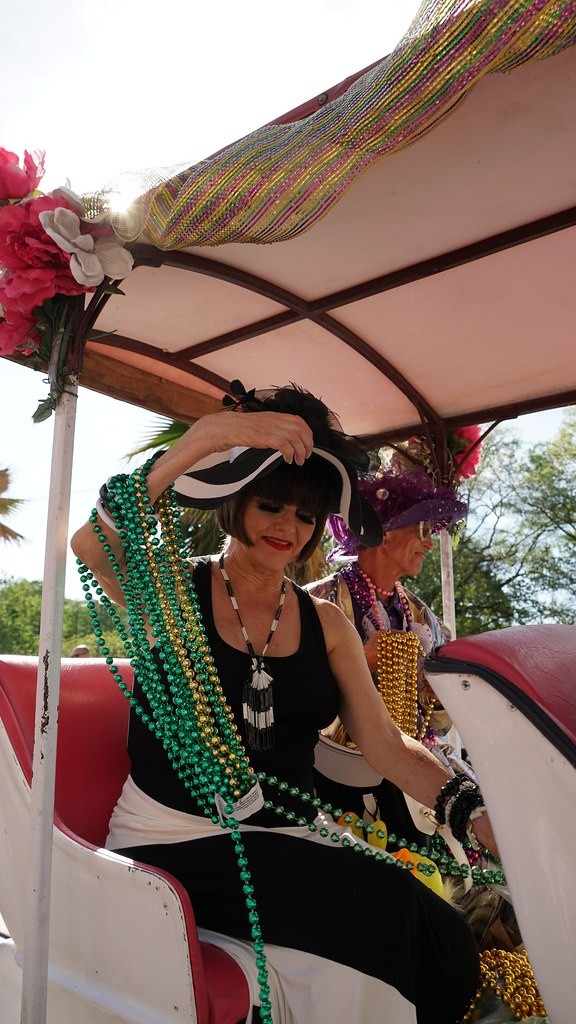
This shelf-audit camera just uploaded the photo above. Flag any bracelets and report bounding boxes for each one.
[434,774,486,848]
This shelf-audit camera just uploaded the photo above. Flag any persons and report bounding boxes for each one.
[301,473,477,781]
[70,383,548,1023]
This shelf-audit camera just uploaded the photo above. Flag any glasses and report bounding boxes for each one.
[417,520,435,543]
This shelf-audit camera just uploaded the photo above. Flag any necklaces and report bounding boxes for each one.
[343,564,435,746]
[77,459,524,1023]
[220,554,287,752]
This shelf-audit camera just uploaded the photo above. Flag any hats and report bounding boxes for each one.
[153,388,387,547]
[327,472,466,568]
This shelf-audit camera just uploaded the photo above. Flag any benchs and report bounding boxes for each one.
[422,624,576,1024]
[0,656,250,1024]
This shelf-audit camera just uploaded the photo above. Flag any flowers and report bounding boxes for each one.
[409,421,483,478]
[0,146,134,423]
